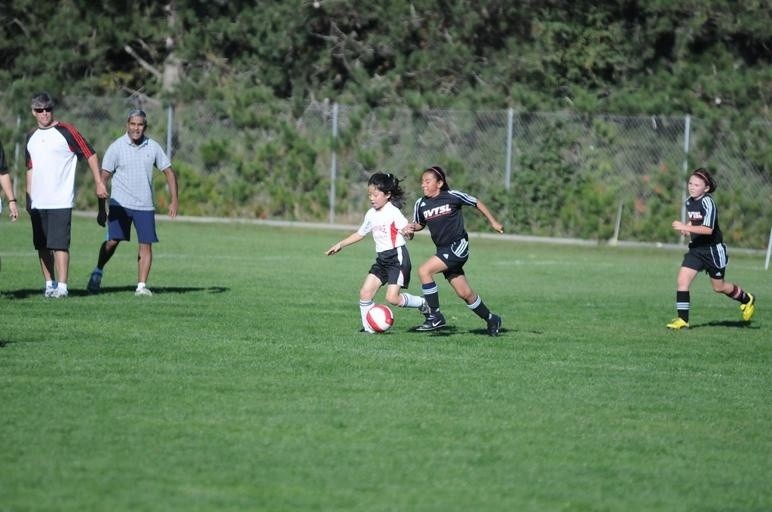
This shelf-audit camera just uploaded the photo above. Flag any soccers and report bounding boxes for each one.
[365,303,394,334]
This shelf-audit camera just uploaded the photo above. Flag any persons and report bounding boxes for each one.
[666,167,757,329]
[406,166,507,336]
[324,172,436,334]
[24,92,108,299]
[88,108,180,296]
[0,141,19,347]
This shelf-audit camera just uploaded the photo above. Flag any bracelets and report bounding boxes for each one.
[6,198,16,203]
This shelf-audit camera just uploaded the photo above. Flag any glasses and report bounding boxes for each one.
[34,107,51,112]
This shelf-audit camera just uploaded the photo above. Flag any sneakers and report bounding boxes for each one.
[51,288,68,297]
[415,298,446,331]
[135,287,152,296]
[740,293,755,321]
[45,286,54,297]
[88,272,102,292]
[666,317,689,329]
[488,314,501,336]
